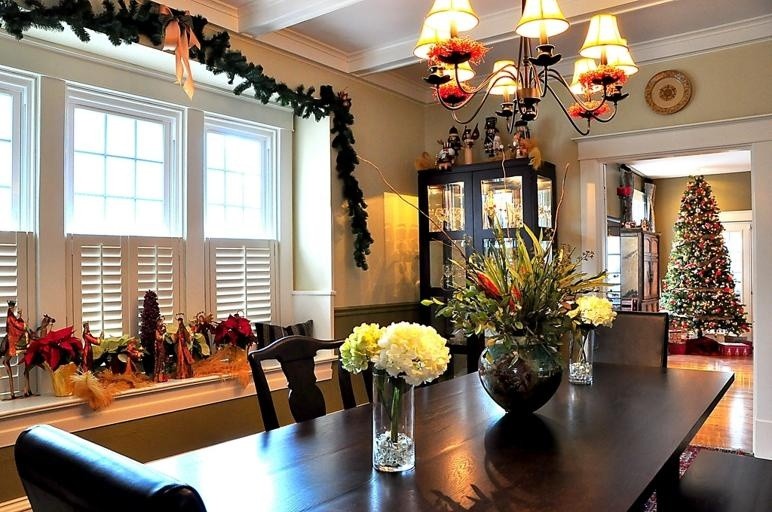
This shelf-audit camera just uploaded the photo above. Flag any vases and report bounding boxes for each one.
[479,339,562,413]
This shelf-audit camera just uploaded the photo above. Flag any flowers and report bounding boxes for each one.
[571,295,617,364]
[339,322,452,444]
[357,156,611,354]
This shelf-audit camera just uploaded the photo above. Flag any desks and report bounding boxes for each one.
[142,368,737,512]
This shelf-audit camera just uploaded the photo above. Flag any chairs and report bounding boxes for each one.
[245,336,355,432]
[594,314,669,367]
[13,425,205,512]
[674,451,772,512]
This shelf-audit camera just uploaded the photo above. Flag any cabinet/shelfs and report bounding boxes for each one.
[419,158,558,380]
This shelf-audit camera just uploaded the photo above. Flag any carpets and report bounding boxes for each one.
[645,443,756,512]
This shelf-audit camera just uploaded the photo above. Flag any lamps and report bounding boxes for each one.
[414,0,639,137]
